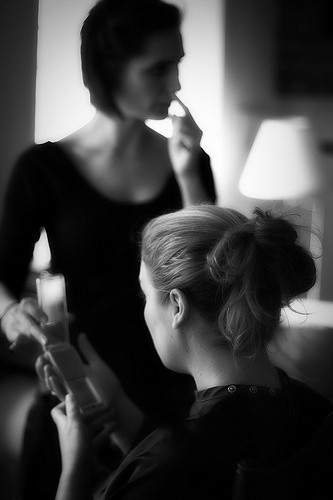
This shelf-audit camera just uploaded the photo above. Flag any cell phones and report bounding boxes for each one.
[46,344,103,417]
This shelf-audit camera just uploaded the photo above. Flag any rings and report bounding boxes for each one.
[51,390,57,396]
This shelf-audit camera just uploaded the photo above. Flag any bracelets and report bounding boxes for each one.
[0,301,17,320]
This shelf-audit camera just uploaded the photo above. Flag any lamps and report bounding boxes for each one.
[239,117,333,329]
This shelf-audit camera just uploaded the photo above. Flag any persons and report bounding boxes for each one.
[37,204,333,500]
[0,0,223,500]
